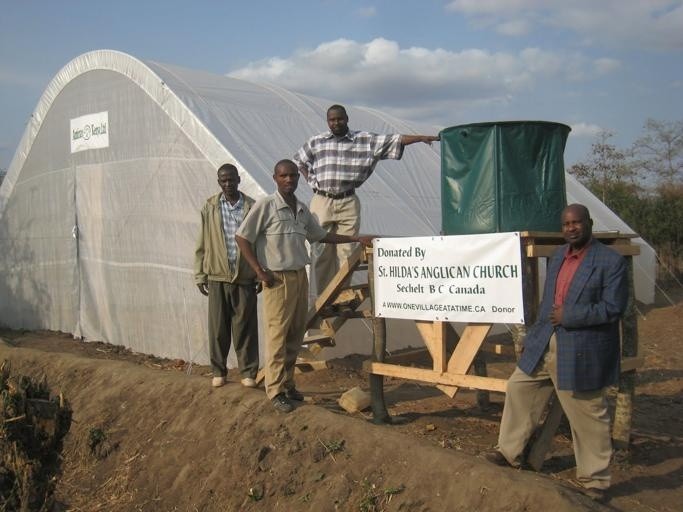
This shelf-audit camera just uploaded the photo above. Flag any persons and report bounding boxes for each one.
[290,103,441,315]
[193,163,262,387]
[234,158,379,413]
[482,204,629,503]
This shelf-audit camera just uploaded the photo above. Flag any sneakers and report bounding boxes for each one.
[286,388,305,401]
[241,377,257,388]
[212,375,226,387]
[270,392,294,413]
[586,486,608,502]
[478,451,520,469]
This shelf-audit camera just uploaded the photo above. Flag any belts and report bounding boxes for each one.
[314,188,356,200]
